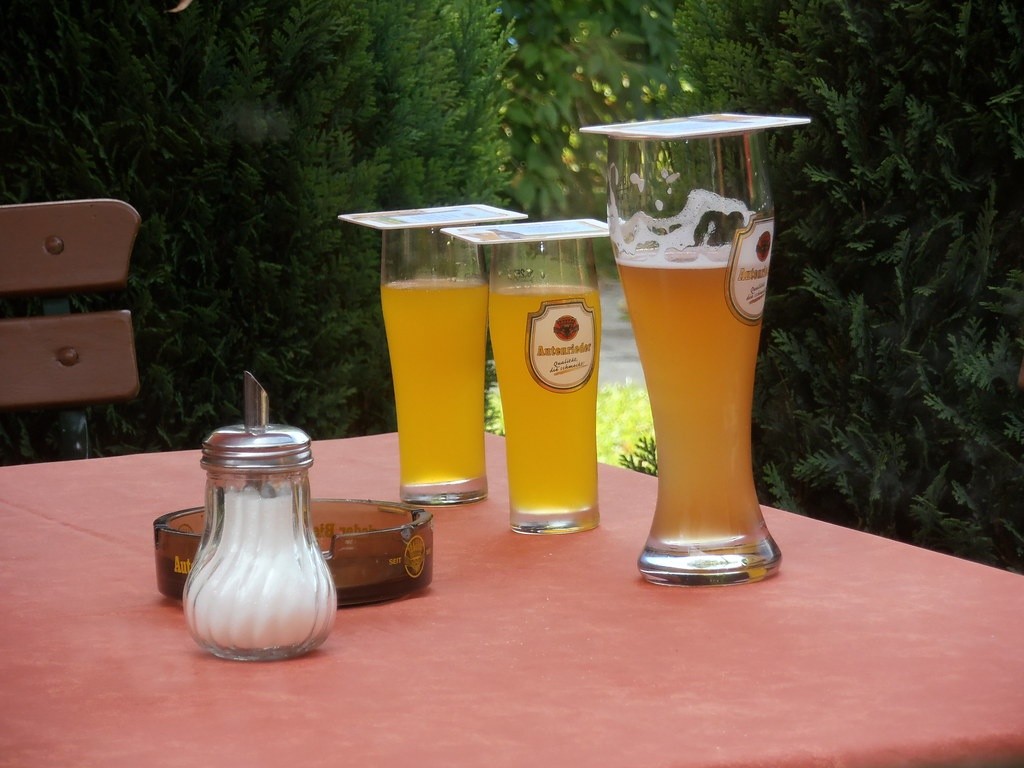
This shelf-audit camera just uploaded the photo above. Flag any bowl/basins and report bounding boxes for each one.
[152,500,437,607]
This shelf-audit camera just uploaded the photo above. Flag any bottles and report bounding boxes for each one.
[182,372,336,660]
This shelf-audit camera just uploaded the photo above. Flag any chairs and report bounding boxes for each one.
[0,197,145,466]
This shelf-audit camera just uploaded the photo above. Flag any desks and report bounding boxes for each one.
[0,417,1023,767]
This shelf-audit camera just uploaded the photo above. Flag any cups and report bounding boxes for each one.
[488,236,599,536]
[380,226,487,503]
[606,128,782,587]
